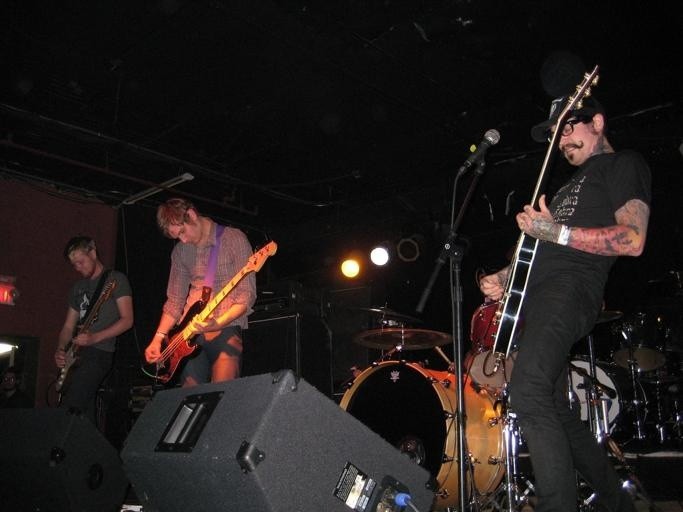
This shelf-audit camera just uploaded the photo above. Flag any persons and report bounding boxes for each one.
[0,367,32,408]
[53,236,133,415]
[144,195,257,388]
[477,91,653,511]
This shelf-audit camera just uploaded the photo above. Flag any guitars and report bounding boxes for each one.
[139,239,279,384]
[492,61,602,360]
[54,278,117,393]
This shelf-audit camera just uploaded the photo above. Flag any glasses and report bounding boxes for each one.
[547,120,579,142]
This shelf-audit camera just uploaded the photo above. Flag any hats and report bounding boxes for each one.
[531,93,604,142]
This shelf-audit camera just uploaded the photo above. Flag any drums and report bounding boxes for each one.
[644,373,677,404]
[561,361,647,442]
[466,348,508,389]
[338,357,507,507]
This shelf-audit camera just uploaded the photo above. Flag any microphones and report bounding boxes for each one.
[458,128,501,173]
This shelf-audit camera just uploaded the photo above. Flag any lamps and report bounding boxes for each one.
[335,231,426,284]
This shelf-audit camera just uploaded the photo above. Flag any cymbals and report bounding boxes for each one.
[355,306,416,322]
[612,346,665,372]
[351,329,452,352]
[596,311,622,324]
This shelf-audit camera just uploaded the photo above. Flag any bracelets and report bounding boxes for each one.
[558,224,571,247]
[156,330,167,338]
[57,348,64,351]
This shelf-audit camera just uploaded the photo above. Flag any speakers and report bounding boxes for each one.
[119,371,436,512]
[0,406,130,512]
